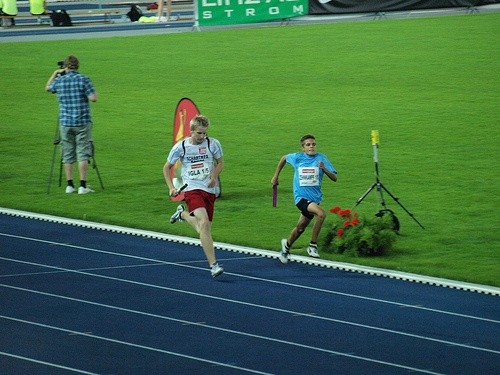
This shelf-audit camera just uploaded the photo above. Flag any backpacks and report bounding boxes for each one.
[127,6,143,22]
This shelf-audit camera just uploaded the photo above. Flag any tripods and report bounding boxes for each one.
[46,118,104,194]
[349,130,425,232]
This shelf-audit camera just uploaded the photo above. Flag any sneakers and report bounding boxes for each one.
[66,185,76,194]
[278,239,291,265]
[210,261,224,278]
[306,246,320,258]
[78,186,95,195]
[170,204,185,224]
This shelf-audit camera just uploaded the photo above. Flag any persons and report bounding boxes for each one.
[271,134,338,264]
[162,114,225,278]
[29,0,47,25]
[0,0,20,27]
[44,56,97,195]
